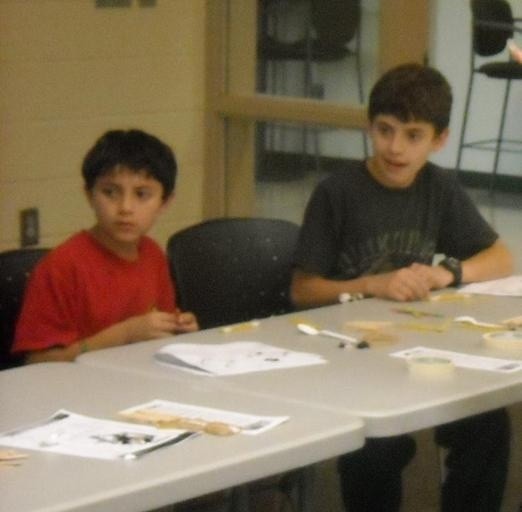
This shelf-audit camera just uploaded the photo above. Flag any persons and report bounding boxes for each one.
[282,62,522,510]
[14,128,289,512]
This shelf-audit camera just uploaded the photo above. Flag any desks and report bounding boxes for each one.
[74,275,522,511]
[0,362,364,511]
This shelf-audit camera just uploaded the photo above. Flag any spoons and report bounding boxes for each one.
[299,323,358,346]
[455,316,509,332]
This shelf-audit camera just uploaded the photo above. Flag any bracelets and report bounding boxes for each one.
[439,256,463,289]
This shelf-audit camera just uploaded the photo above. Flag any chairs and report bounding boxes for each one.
[455,0,522,200]
[257,0,369,175]
[0,248,55,368]
[167,214,313,336]
